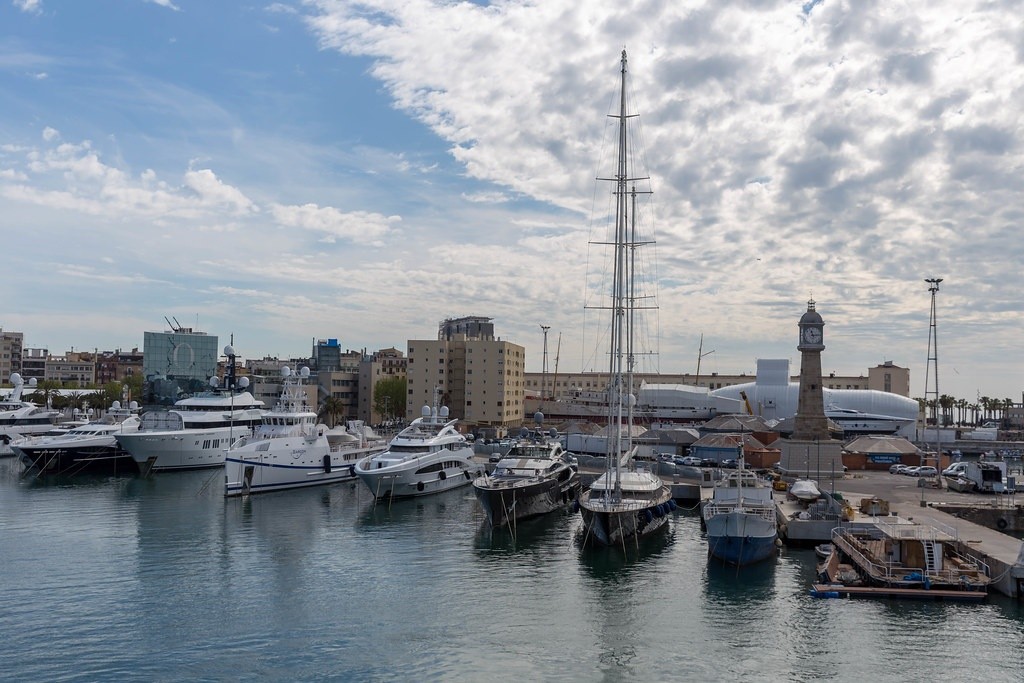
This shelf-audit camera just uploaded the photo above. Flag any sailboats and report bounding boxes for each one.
[577,42,678,548]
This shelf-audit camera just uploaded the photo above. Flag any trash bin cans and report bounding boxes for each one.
[673,474,680,484]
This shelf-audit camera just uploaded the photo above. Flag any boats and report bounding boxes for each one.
[703,440,778,567]
[0,386,89,460]
[223,367,392,498]
[354,405,486,501]
[113,390,270,474]
[471,412,582,530]
[10,422,138,476]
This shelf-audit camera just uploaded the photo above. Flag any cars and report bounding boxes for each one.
[682,456,702,467]
[889,464,908,475]
[670,454,684,465]
[656,452,669,463]
[701,458,719,468]
[902,465,918,474]
[909,465,939,479]
[460,429,517,448]
[719,458,738,469]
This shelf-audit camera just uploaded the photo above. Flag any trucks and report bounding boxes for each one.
[559,433,617,457]
